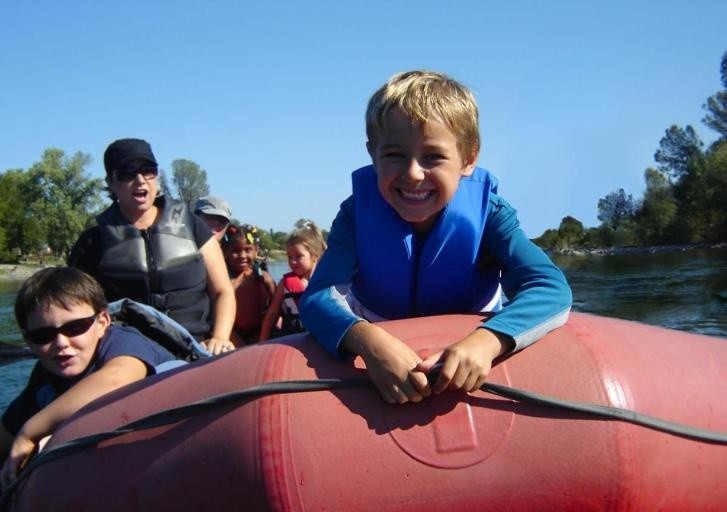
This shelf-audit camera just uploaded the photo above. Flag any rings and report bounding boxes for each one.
[221,345,229,351]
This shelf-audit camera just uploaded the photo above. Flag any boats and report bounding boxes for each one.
[14,311,726,511]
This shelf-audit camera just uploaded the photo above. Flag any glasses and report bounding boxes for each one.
[19,311,100,349]
[114,163,157,182]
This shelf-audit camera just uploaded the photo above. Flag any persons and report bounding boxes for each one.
[296,69,575,405]
[190,195,232,245]
[0,262,214,493]
[66,137,239,360]
[220,224,275,350]
[259,217,329,343]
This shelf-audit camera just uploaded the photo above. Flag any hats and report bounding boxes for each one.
[102,138,158,179]
[194,195,233,228]
